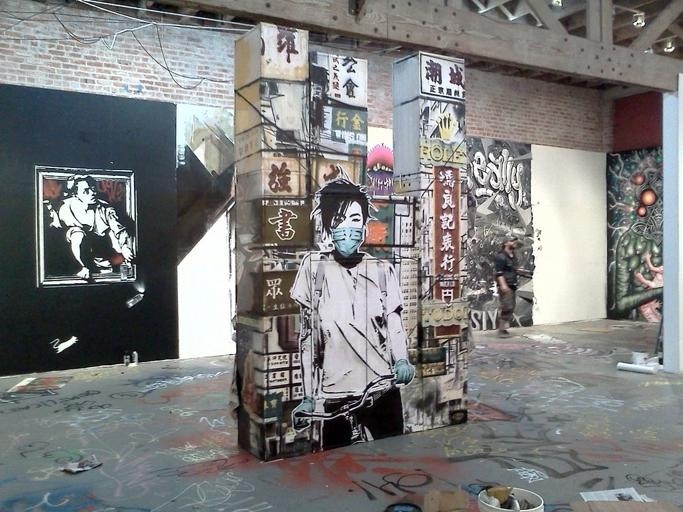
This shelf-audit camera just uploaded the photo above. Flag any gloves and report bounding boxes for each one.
[392,358,417,386]
[290,396,317,433]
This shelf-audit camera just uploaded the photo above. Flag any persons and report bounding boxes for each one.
[289,178,415,452]
[42,174,134,279]
[495,236,517,338]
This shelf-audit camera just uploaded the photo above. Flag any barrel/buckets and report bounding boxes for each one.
[630,350,650,366]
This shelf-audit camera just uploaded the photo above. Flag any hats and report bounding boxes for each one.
[501,234,521,244]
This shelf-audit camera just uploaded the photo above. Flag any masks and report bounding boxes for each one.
[326,224,365,259]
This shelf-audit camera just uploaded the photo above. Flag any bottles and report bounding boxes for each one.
[131,350,138,365]
[125,293,143,308]
[119,261,128,280]
[123,351,130,366]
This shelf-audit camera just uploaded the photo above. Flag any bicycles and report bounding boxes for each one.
[291,371,399,447]
